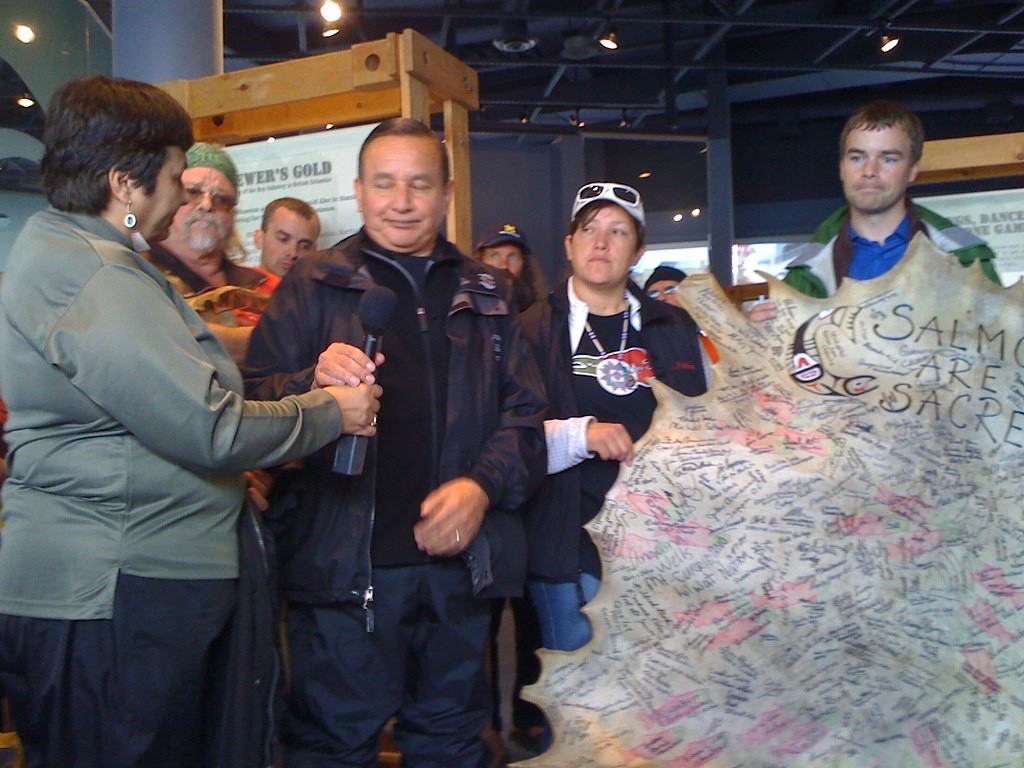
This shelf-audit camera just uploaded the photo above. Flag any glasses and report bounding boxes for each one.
[649,285,680,299]
[183,187,238,214]
[572,183,645,224]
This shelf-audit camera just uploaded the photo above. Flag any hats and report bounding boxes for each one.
[184,142,241,191]
[477,224,530,252]
[643,265,686,292]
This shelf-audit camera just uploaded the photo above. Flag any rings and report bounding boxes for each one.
[456,530,460,543]
[370,416,377,426]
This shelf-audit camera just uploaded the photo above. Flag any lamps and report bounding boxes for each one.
[491,19,539,53]
[15,92,35,109]
[599,31,619,50]
[877,30,900,52]
[318,0,344,38]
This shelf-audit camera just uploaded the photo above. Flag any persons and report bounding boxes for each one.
[1,76,720,767]
[747,101,1006,322]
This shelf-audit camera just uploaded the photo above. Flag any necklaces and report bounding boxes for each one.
[584,293,638,396]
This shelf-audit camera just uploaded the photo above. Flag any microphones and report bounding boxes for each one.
[333,284,396,476]
